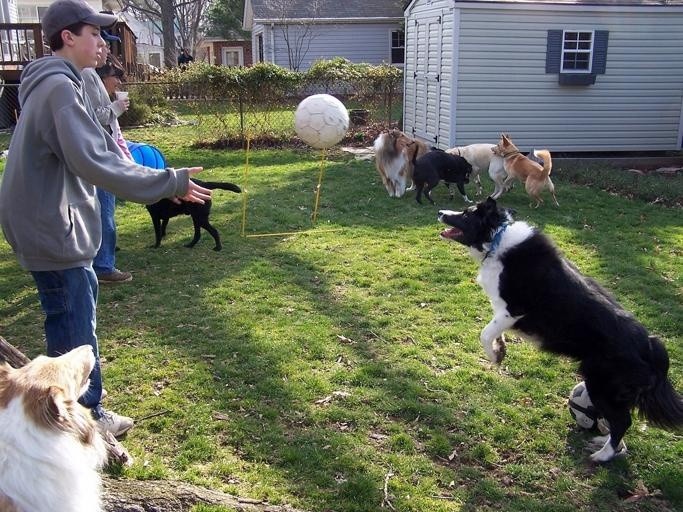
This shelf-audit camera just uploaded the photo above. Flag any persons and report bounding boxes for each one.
[96,51,137,251]
[0,0,213,436]
[177,48,194,66]
[80,29,133,284]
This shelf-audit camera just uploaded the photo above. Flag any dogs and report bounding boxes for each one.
[373,127,428,198]
[445,143,507,200]
[438,195,683,463]
[0,343,110,512]
[146,177,241,251]
[490,133,559,209]
[412,143,473,205]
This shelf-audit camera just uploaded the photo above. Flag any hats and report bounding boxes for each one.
[42,1,121,40]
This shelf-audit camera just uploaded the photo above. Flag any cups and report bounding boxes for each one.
[114,91,128,102]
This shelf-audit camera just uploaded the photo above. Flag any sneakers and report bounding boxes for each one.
[98,269,133,284]
[96,411,133,437]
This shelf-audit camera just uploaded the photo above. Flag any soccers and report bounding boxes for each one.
[294,94,349,149]
